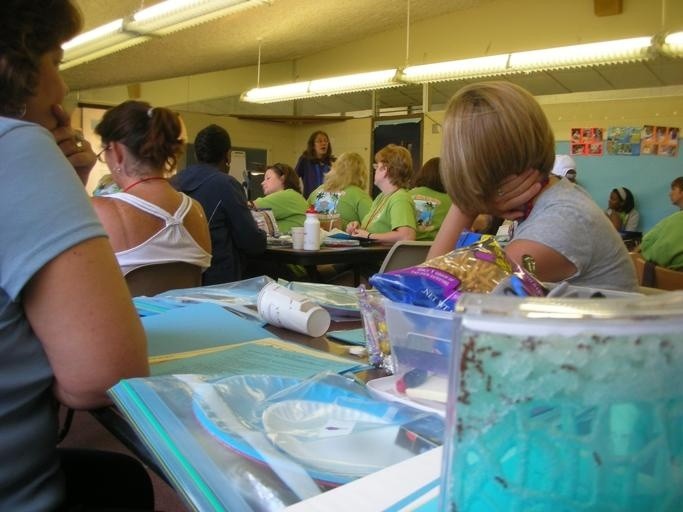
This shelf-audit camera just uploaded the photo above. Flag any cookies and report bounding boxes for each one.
[425,250,500,294]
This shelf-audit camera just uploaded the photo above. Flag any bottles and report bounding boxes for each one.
[302,204,320,253]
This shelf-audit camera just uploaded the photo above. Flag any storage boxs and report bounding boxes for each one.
[379,285,683,512]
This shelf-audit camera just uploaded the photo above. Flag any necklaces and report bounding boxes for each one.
[364,188,399,231]
[122,176,166,194]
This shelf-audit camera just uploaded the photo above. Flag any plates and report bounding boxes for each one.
[191,372,444,489]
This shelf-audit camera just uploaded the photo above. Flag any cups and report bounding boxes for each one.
[290,227,304,251]
[257,282,332,338]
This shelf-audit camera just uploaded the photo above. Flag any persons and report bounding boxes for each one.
[307,152,374,234]
[168,124,267,286]
[551,155,576,182]
[407,157,452,241]
[567,125,681,157]
[85,97,214,293]
[1,1,165,512]
[603,188,639,252]
[294,131,337,200]
[636,176,682,272]
[346,143,415,243]
[425,82,639,293]
[247,163,310,235]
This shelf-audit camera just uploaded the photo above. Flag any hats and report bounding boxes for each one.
[550,155,577,178]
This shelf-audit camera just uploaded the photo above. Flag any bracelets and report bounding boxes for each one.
[367,233,371,240]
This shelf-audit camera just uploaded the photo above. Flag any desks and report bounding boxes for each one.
[57,235,564,512]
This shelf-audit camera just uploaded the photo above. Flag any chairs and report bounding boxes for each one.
[629,250,683,294]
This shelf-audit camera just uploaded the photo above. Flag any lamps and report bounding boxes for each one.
[124,2,268,38]
[57,18,151,70]
[397,34,664,84]
[662,30,682,60]
[240,64,404,105]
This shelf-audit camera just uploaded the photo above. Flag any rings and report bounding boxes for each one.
[496,187,503,196]
[73,139,83,151]
